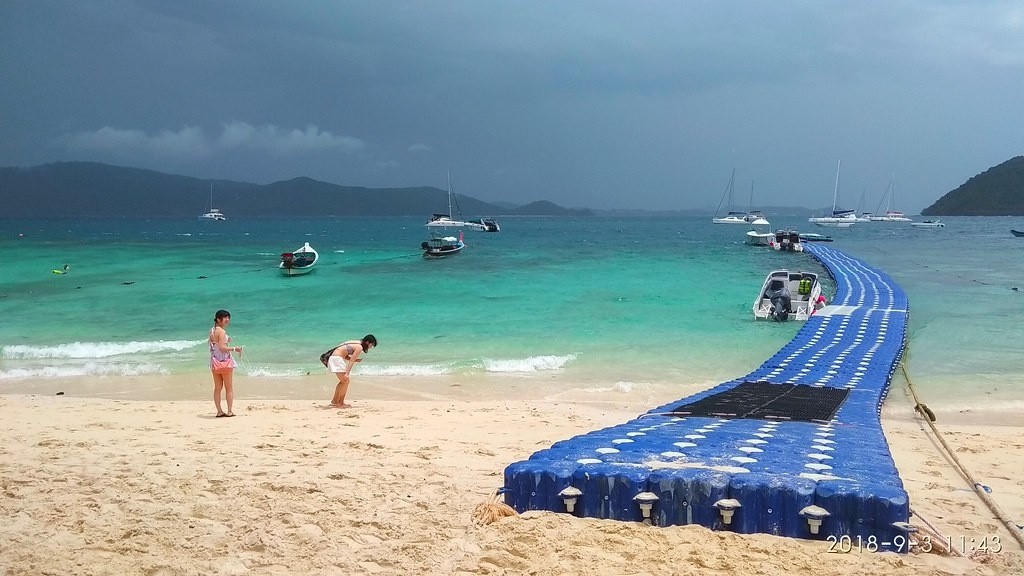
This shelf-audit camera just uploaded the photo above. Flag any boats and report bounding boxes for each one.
[278,242,319,276]
[753,268,821,322]
[1010,229,1024,237]
[909,219,946,229]
[420,230,467,259]
[464,216,501,233]
[746,217,832,251]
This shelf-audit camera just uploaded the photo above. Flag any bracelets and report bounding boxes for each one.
[233,347,235,352]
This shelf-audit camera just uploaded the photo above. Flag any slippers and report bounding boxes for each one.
[216,413,237,418]
[331,402,351,409]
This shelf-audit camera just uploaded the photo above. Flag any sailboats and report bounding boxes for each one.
[808,160,913,228]
[424,168,466,227]
[711,168,767,224]
[196,182,227,221]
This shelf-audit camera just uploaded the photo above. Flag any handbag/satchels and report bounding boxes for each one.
[211,355,239,372]
[319,342,362,366]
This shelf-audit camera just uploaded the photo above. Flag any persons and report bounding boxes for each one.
[328,334,377,408]
[208,310,242,417]
